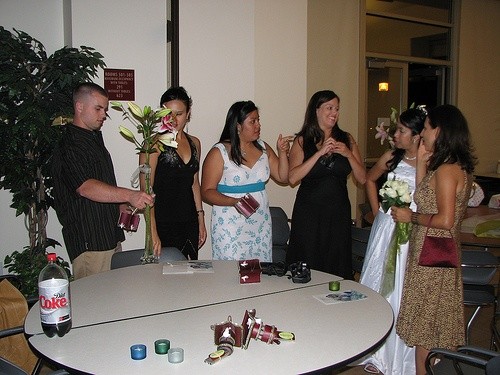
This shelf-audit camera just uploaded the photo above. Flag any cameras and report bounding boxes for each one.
[291,261,311,283]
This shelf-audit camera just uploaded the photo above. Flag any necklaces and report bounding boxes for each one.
[404,154,416,160]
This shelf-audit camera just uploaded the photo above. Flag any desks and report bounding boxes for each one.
[23,261,394,375]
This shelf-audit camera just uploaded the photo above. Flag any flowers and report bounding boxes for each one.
[110,100,179,263]
[378,179,415,245]
[375,103,428,151]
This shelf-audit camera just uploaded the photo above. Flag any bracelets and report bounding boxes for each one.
[411,212,421,225]
[197,210,204,216]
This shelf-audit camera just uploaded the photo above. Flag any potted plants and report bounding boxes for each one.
[0,26,106,311]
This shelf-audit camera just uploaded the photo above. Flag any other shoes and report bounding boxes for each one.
[363,362,380,374]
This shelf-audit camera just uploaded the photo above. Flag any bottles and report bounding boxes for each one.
[38,253,72,338]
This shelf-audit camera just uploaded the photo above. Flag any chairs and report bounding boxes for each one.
[352,227,370,275]
[425,345,500,375]
[0,278,70,375]
[460,242,500,284]
[463,284,500,352]
[269,207,292,262]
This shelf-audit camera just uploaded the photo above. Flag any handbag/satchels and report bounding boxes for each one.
[418,214,459,268]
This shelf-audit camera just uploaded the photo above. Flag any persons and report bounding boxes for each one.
[138,86,207,259]
[201,101,296,263]
[390,104,479,375]
[53,83,156,280]
[289,91,367,280]
[347,107,440,375]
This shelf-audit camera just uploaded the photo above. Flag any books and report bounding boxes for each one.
[163,262,193,274]
[187,261,214,274]
